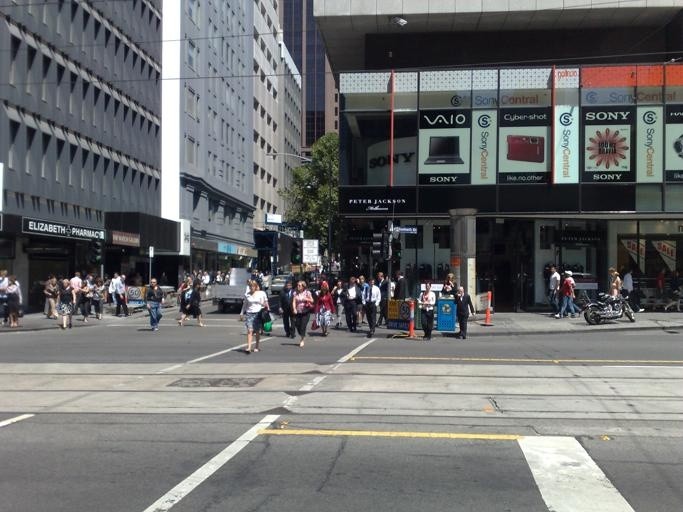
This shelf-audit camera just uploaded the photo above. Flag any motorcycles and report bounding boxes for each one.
[583,286,636,324]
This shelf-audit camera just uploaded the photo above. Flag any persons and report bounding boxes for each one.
[608,266,683,312]
[548,266,583,318]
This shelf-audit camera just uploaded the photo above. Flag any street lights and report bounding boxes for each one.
[265,151,333,277]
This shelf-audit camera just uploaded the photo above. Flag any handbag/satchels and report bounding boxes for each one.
[305,301,314,309]
[311,320,319,330]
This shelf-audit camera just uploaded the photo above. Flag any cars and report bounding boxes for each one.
[270,270,325,294]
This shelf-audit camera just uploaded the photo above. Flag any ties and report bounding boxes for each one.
[369,287,372,304]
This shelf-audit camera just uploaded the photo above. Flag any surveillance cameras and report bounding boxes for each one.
[391,16,408,27]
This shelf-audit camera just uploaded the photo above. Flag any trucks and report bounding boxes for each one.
[205,268,251,313]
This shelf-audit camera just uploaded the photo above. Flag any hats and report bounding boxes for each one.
[565,271,572,276]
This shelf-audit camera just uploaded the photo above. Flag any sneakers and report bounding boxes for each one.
[555,313,563,318]
[82,318,204,331]
[245,340,306,353]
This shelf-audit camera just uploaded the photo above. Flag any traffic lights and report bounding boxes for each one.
[290,238,302,265]
[370,228,390,261]
[391,238,402,261]
[89,238,104,267]
